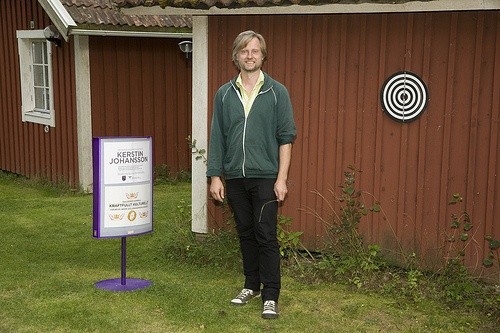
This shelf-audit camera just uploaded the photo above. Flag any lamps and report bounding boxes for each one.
[44,25,61,47]
[178,40,193,60]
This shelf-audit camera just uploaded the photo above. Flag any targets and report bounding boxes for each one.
[379,70,431,123]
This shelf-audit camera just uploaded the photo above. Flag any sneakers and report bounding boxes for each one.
[262,300,278,319]
[231,288,261,306]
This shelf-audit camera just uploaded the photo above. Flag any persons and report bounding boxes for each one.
[208,30,297,318]
